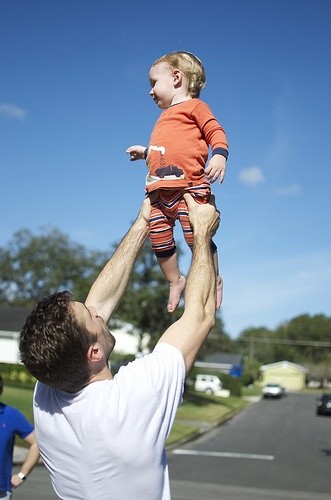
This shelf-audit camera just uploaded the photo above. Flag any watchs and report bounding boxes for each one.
[18,472,27,481]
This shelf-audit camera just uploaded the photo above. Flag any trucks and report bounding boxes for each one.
[188,372,222,395]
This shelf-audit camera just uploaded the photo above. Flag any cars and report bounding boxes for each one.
[316,393,331,416]
[262,382,283,399]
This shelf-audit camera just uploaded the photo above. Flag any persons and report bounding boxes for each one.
[0,377,40,500]
[19,189,221,500]
[126,50,231,313]
